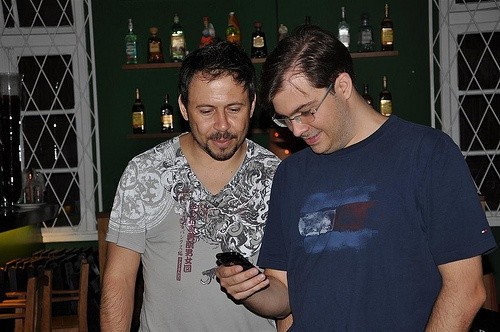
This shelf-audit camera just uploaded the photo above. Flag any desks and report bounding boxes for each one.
[0,203,58,233]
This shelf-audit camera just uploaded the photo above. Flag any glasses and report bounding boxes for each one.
[272,79,336,127]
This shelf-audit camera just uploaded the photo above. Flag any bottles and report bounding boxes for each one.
[380,2,393,51]
[169,12,186,62]
[379,75,393,117]
[146,26,163,64]
[124,17,139,64]
[224,11,241,47]
[23,172,43,204]
[304,16,310,25]
[198,17,213,48]
[160,93,174,131]
[336,5,351,52]
[132,88,145,134]
[252,21,268,59]
[362,81,373,108]
[357,13,375,52]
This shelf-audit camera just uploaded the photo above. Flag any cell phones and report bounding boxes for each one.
[216,251,261,278]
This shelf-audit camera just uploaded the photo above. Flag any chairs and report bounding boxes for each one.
[0,254,91,332]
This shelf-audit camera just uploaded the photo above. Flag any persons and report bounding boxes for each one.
[100,38,281,332]
[216,25,498,332]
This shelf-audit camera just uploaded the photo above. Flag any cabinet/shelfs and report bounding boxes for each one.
[121,47,400,140]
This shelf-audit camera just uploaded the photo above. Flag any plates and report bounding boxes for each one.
[15,204,44,208]
